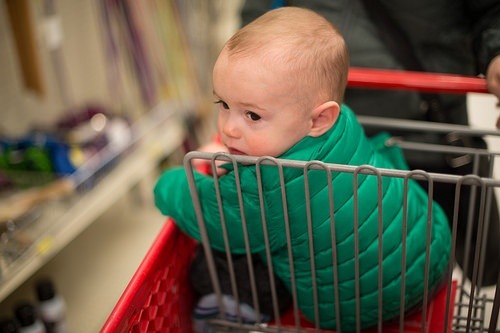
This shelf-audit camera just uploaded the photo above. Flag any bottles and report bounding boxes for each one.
[34,279,68,333]
[13,303,47,333]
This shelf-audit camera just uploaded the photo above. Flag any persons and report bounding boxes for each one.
[240,1,500,288]
[154,7,454,330]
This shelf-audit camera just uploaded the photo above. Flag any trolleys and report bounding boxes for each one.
[100,66,500,333]
